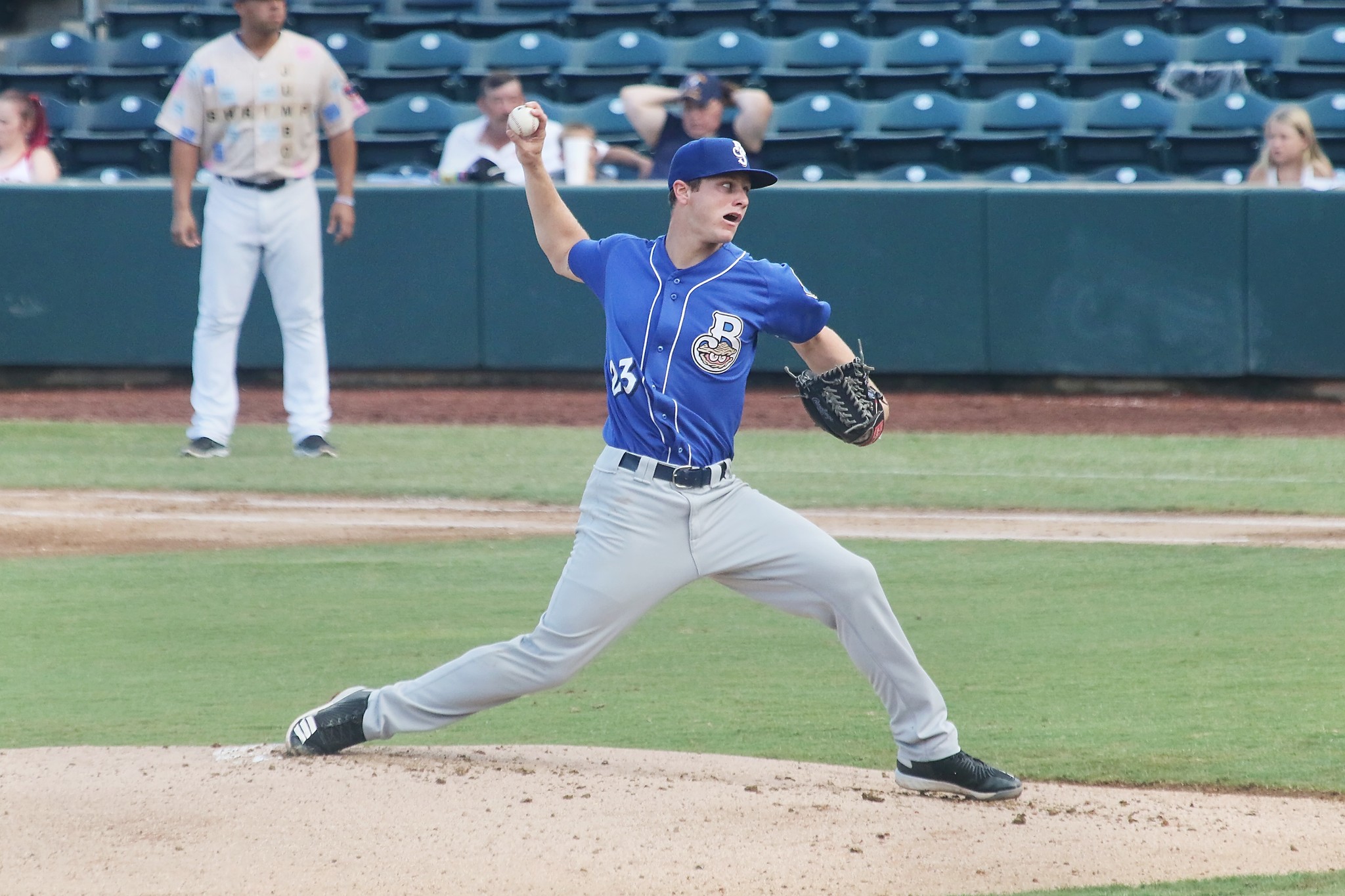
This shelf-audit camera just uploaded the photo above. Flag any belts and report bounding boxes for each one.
[218,176,285,191]
[618,453,726,489]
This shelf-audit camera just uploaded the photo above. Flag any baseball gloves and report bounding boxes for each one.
[796,357,885,448]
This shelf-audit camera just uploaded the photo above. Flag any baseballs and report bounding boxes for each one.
[508,105,540,137]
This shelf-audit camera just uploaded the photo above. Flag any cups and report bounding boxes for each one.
[559,124,595,187]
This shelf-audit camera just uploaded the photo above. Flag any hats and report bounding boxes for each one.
[668,137,778,189]
[673,73,723,105]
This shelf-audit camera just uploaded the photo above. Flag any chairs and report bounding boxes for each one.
[0,0,1345,183]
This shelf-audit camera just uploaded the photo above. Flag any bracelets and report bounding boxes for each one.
[335,194,355,206]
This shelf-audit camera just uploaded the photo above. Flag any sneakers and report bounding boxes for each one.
[293,435,336,457]
[183,438,229,457]
[287,685,372,755]
[895,749,1023,801]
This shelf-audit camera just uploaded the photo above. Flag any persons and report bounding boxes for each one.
[283,102,1025,801]
[158,1,369,459]
[1248,106,1332,181]
[429,69,774,186]
[0,87,63,183]
